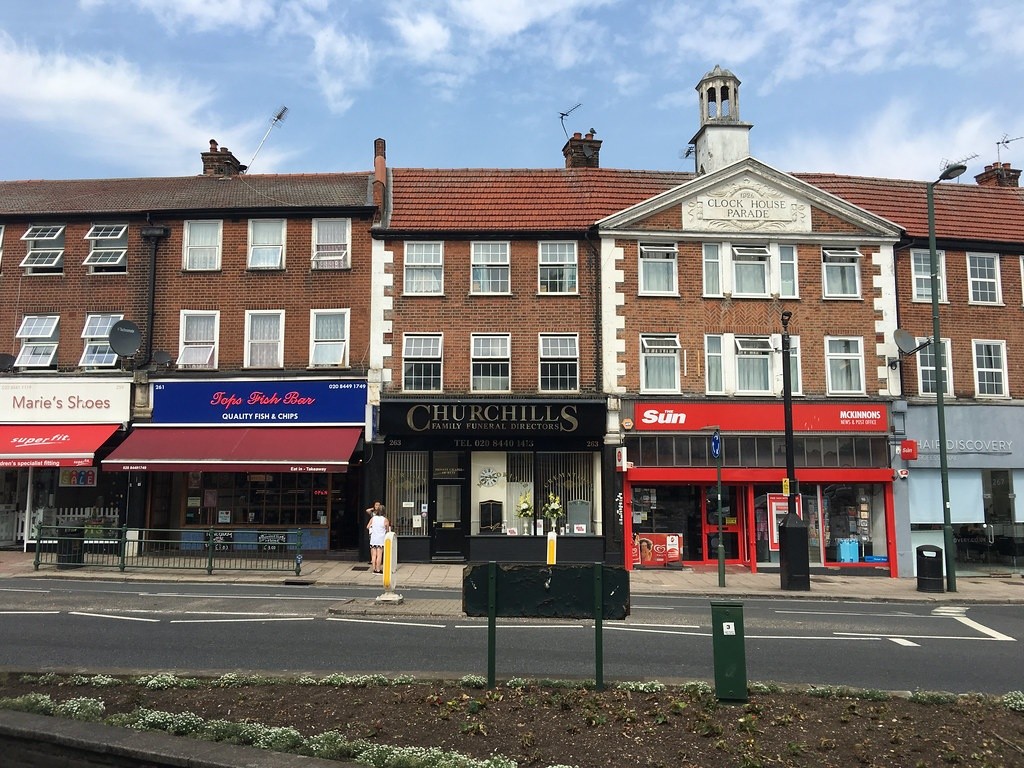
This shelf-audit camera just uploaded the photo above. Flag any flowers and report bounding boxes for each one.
[513,489,535,518]
[542,491,566,519]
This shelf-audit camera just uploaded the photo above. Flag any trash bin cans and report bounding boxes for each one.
[916,545,944,593]
[57,521,85,570]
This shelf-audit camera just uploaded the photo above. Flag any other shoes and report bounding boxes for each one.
[374,569,383,573]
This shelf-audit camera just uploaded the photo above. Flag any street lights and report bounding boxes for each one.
[926,165,968,594]
[781,309,797,510]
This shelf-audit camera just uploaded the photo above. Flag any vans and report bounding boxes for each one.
[708,492,831,527]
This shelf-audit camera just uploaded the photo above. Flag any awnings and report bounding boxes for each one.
[0,423,121,467]
[101,428,366,474]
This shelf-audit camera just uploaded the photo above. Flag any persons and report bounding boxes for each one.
[365,500,389,575]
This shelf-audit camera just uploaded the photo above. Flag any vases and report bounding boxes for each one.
[523,517,529,536]
[551,517,558,535]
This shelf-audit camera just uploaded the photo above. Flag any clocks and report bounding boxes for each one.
[479,468,498,487]
